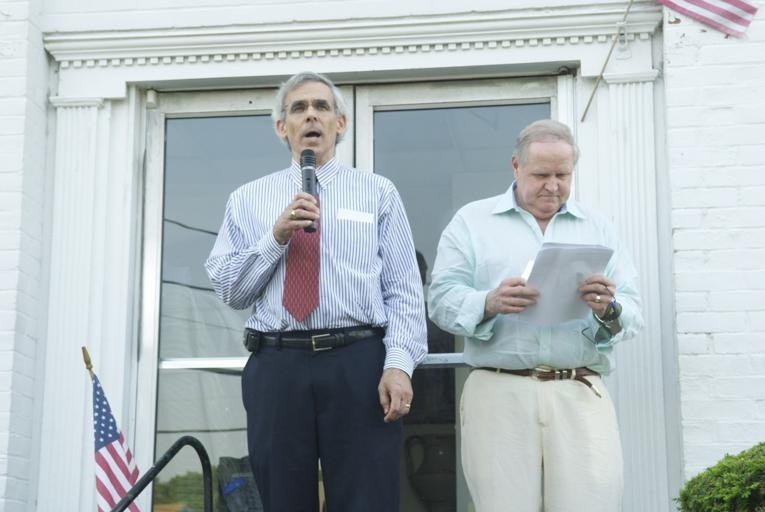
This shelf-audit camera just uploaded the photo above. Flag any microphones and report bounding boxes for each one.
[299,148,319,234]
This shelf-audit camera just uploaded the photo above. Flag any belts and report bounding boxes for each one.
[240,322,384,353]
[477,367,609,401]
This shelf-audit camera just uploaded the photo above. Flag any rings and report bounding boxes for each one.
[405,403,412,408]
[291,210,296,220]
[595,295,601,303]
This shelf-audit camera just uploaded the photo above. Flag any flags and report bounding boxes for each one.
[650,1,759,38]
[92,370,141,512]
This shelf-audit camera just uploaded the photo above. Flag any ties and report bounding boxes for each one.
[281,175,321,325]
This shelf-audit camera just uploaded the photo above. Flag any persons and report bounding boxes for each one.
[427,117,646,512]
[200,66,428,512]
